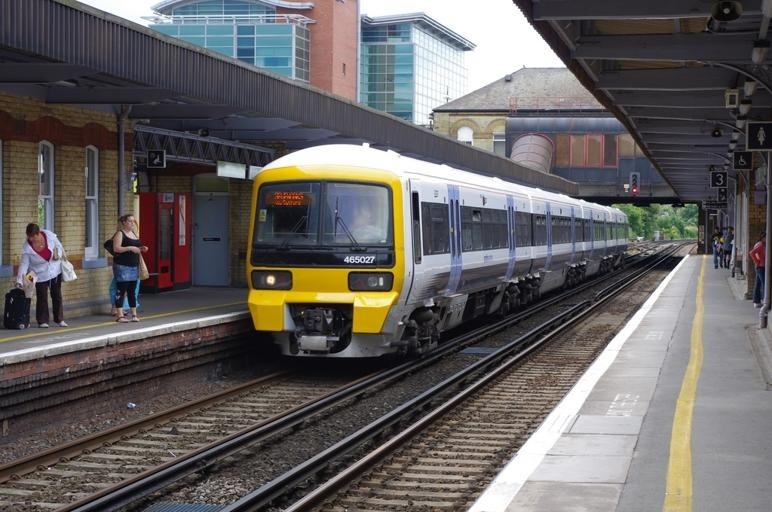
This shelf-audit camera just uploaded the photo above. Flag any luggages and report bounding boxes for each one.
[4,288,31,329]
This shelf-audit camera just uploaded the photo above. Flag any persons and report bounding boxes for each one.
[15,223,68,328]
[711,226,734,269]
[109,214,149,322]
[749,232,766,308]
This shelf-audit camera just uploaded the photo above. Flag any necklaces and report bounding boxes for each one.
[35,237,43,249]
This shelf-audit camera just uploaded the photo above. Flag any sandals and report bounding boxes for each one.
[113,312,140,323]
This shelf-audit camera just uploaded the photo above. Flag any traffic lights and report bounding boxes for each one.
[630,172,640,194]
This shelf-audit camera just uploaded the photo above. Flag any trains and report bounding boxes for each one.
[246,142,629,361]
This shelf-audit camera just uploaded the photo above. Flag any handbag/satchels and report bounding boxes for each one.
[139,253,149,280]
[103,230,122,256]
[61,246,78,282]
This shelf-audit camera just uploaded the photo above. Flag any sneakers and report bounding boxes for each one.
[111,308,128,316]
[753,303,762,308]
[57,320,68,327]
[39,322,49,328]
